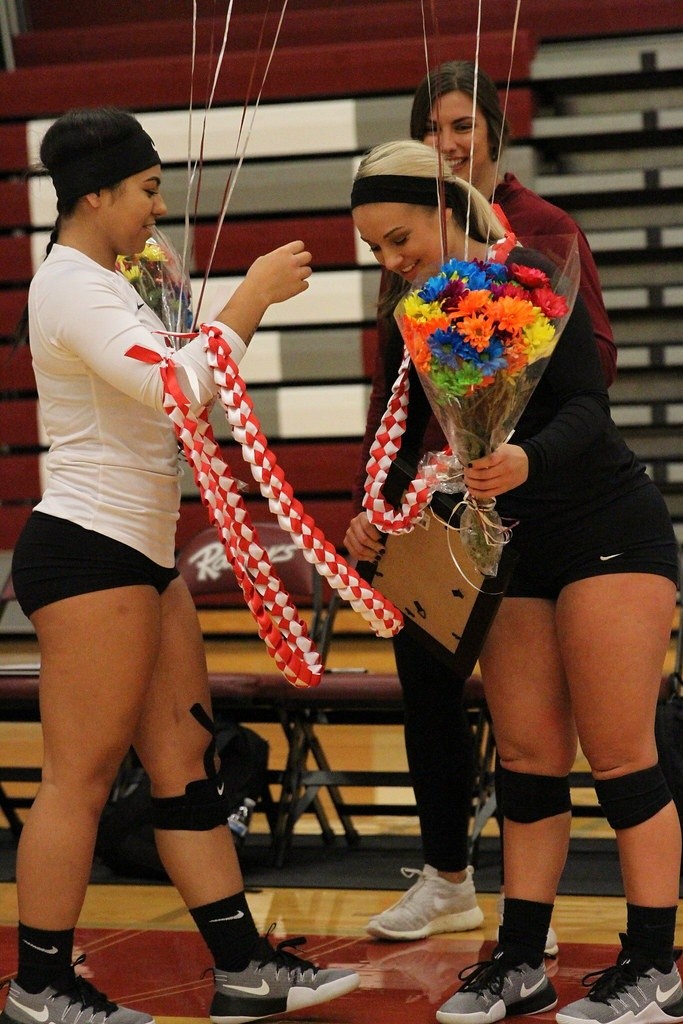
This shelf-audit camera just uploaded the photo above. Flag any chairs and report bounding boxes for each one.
[1,519,683,878]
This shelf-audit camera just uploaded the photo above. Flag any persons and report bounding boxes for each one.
[343,139,683,1024]
[364,60,618,956]
[0,106,358,1024]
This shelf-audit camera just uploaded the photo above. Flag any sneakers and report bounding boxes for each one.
[497,899,560,958]
[553,933,682,1024]
[435,945,559,1023]
[0,953,156,1024]
[365,865,485,939]
[196,922,362,1023]
